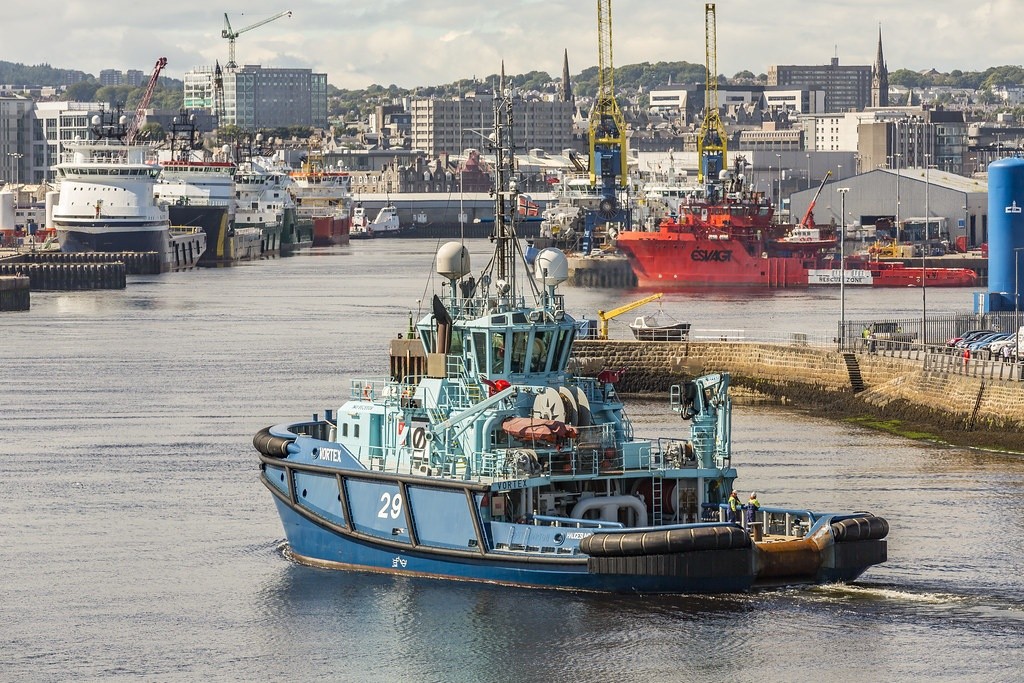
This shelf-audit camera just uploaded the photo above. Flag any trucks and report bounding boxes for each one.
[860,321,918,350]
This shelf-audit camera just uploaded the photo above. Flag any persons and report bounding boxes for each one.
[745,492,760,531]
[727,490,740,524]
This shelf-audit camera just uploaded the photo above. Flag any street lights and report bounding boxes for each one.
[806,153,811,189]
[924,154,933,242]
[895,153,903,244]
[838,164,842,182]
[776,153,782,225]
[1013,248,1023,362]
[837,188,851,351]
[8,153,24,209]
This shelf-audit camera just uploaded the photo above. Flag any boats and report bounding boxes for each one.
[153,106,238,270]
[627,314,693,342]
[51,100,174,274]
[253,63,889,601]
[609,170,978,292]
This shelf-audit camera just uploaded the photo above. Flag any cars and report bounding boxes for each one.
[950,329,1024,361]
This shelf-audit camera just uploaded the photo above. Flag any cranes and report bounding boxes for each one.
[696,3,733,205]
[586,1,631,194]
[221,9,293,72]
